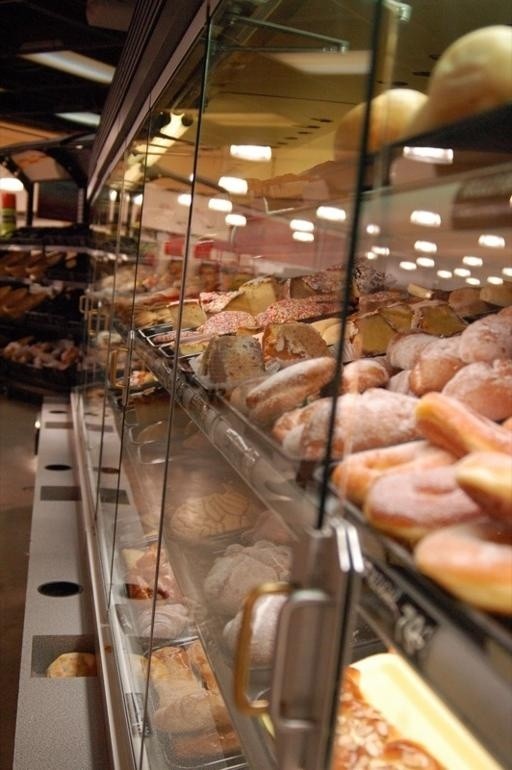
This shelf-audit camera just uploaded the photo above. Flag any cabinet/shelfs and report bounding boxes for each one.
[0,0,512,770]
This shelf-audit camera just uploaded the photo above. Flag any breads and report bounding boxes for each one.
[46,261,512,770]
